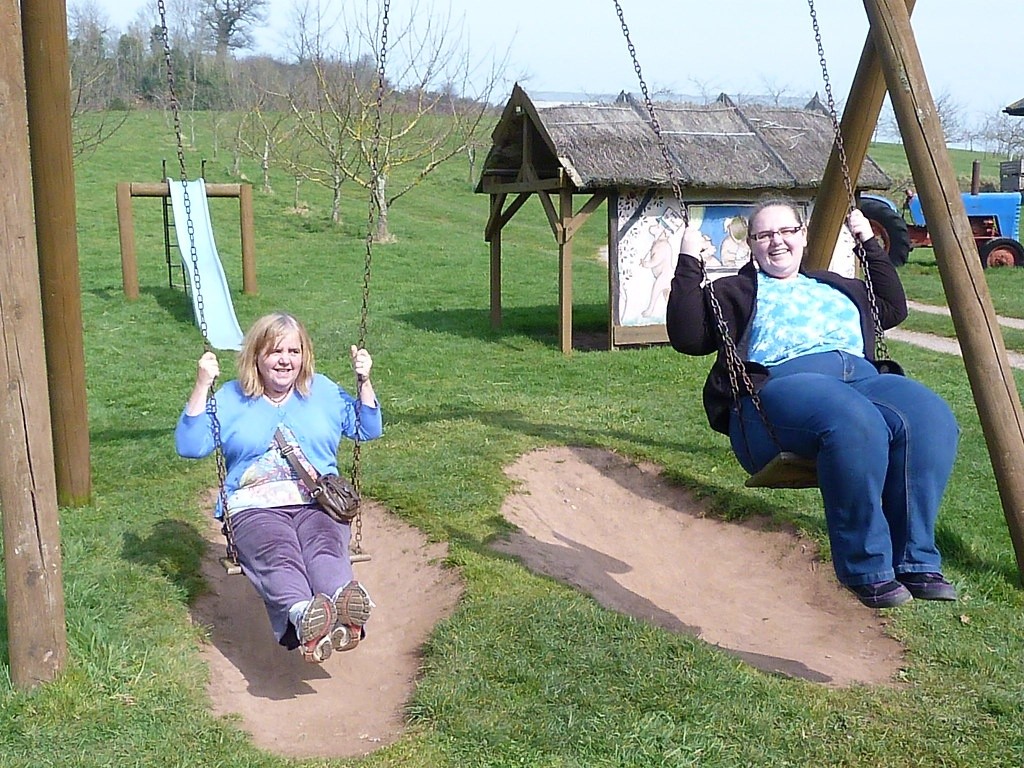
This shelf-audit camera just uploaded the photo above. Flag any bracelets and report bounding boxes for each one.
[357,378,370,394]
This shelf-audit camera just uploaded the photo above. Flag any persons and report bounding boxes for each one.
[665,191,964,611]
[171,312,384,664]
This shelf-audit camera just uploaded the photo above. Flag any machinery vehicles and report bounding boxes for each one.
[855,160,1024,268]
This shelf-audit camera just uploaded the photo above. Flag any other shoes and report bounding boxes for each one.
[296,592,337,664]
[332,580,376,651]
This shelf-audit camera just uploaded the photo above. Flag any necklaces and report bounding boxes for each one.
[261,383,294,409]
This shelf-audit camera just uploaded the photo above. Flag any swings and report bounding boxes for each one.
[158,0,393,577]
[613,0,906,488]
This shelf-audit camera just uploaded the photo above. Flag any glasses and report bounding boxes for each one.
[750,224,804,244]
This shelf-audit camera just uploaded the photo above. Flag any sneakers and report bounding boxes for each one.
[895,568,956,602]
[845,577,913,608]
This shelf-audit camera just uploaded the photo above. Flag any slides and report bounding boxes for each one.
[166,177,250,352]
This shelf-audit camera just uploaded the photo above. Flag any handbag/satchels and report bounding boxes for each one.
[309,472,360,523]
